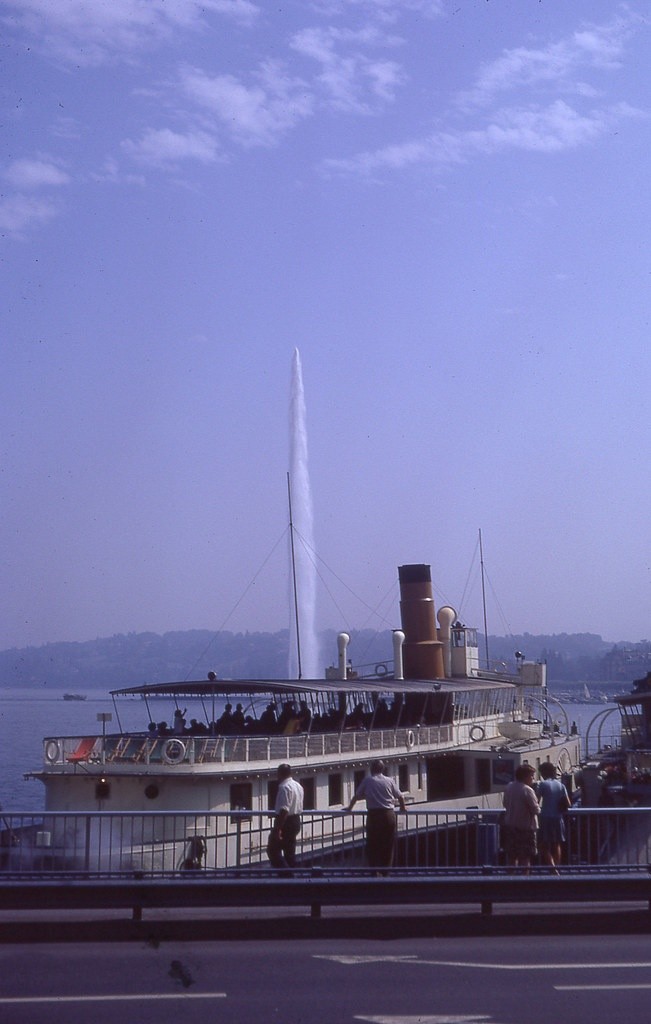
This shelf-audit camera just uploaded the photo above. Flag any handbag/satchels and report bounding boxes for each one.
[560,797,570,813]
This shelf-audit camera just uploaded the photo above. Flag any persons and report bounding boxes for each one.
[537,762,571,876]
[266,763,303,878]
[341,760,408,878]
[141,698,427,748]
[503,765,541,876]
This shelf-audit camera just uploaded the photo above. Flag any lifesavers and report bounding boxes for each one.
[406,731,416,749]
[554,712,566,728]
[43,741,60,761]
[160,740,186,764]
[468,727,486,742]
[374,664,389,677]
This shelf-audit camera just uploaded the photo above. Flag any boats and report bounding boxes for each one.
[63,692,87,701]
[16,348,586,876]
